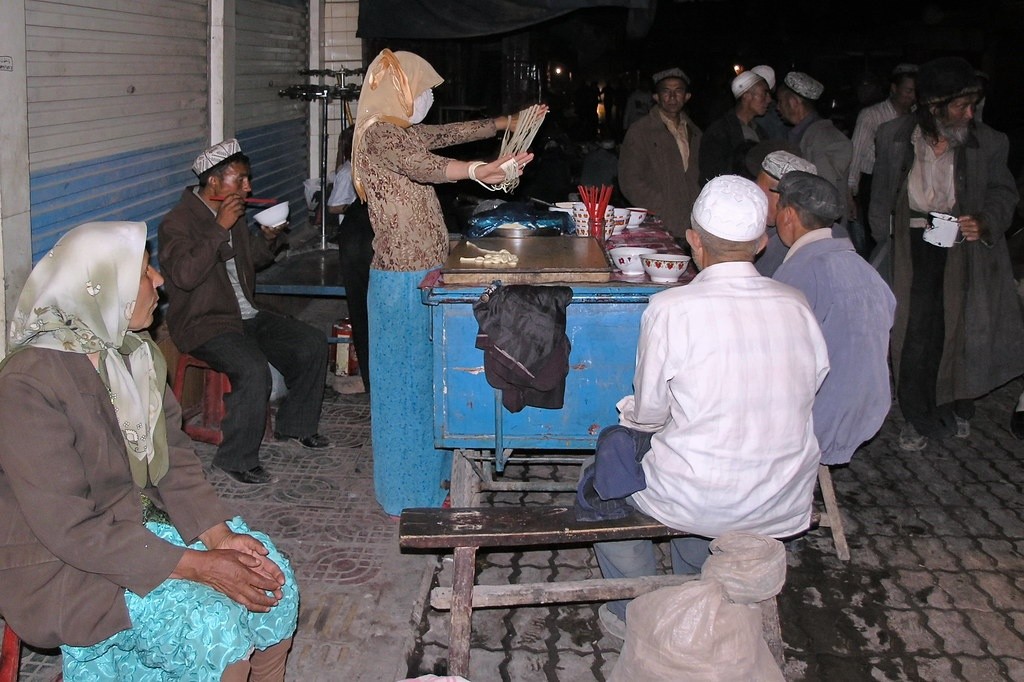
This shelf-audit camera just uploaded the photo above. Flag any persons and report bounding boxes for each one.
[158,138,332,485]
[579,175,829,640]
[619,55,1024,465]
[574,81,617,123]
[0,220,299,682]
[327,48,548,517]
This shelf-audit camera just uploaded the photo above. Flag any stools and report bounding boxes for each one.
[0,621,23,682]
[169,353,273,447]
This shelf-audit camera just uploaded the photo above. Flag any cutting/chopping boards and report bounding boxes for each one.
[440,235,612,284]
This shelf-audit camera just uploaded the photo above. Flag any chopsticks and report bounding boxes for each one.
[209,195,277,203]
[577,184,613,217]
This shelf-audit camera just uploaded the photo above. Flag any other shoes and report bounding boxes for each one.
[1010,400,1024,440]
[951,415,970,438]
[599,603,629,640]
[899,419,928,451]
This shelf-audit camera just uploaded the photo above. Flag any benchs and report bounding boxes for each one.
[813,461,851,562]
[400,507,785,682]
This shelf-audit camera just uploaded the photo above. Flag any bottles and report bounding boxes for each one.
[588,217,605,246]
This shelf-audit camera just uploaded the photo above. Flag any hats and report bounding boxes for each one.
[750,65,776,90]
[693,175,769,242]
[191,138,244,178]
[651,65,691,86]
[731,70,764,99]
[784,72,824,101]
[912,57,982,103]
[761,149,818,182]
[887,62,921,84]
[769,170,846,220]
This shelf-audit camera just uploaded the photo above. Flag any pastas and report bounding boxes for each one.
[470,105,546,195]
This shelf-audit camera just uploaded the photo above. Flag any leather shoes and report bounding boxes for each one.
[274,428,337,451]
[211,460,279,486]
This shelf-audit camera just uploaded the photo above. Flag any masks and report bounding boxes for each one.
[408,92,434,125]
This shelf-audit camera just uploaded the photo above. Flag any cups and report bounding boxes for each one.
[922,211,966,247]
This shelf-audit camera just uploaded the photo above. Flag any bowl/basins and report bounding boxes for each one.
[253,201,289,228]
[638,253,692,283]
[549,201,649,242]
[467,219,561,238]
[609,247,656,275]
